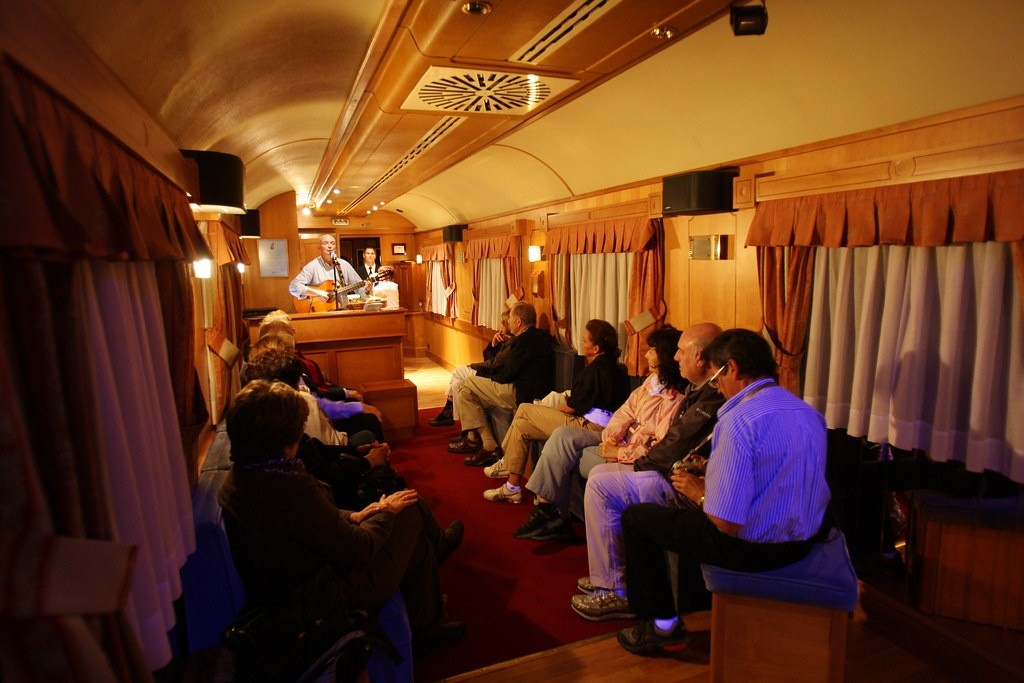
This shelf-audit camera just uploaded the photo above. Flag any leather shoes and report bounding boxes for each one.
[448,436,480,453]
[429,413,453,426]
[464,446,503,464]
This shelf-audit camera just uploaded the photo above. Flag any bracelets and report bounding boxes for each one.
[697,495,705,507]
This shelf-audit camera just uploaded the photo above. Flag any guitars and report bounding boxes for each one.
[293,269,393,313]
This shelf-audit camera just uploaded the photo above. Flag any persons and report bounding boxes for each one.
[615,328,830,658]
[218,308,468,651]
[288,234,366,313]
[512,325,689,542]
[428,301,557,466]
[482,317,630,504]
[354,246,380,282]
[570,323,727,623]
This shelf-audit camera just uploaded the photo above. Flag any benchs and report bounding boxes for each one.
[182,420,415,683]
[487,346,645,520]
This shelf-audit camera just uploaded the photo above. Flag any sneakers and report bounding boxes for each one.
[618,616,687,652]
[483,457,510,478]
[577,577,608,594]
[482,483,522,503]
[571,591,638,621]
[514,507,561,538]
[531,518,573,540]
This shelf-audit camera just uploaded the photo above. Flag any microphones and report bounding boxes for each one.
[331,251,337,267]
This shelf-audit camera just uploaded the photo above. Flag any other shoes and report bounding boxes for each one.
[414,621,468,651]
[435,520,463,565]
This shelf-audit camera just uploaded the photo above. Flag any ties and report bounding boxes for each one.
[369,266,373,275]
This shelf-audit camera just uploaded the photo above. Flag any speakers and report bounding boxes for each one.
[182,148,246,215]
[662,169,735,217]
[241,209,260,239]
[443,224,467,243]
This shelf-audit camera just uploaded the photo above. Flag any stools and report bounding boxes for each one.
[702,529,858,683]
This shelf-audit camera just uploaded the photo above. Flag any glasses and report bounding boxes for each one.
[707,361,728,388]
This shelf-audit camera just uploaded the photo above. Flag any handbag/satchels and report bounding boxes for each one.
[224,595,317,683]
